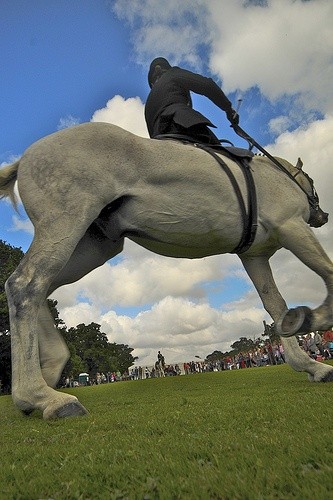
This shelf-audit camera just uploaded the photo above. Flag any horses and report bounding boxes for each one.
[155,360,166,378]
[0,121,333,420]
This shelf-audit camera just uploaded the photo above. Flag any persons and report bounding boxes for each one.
[63,328,333,385]
[145,57,239,145]
